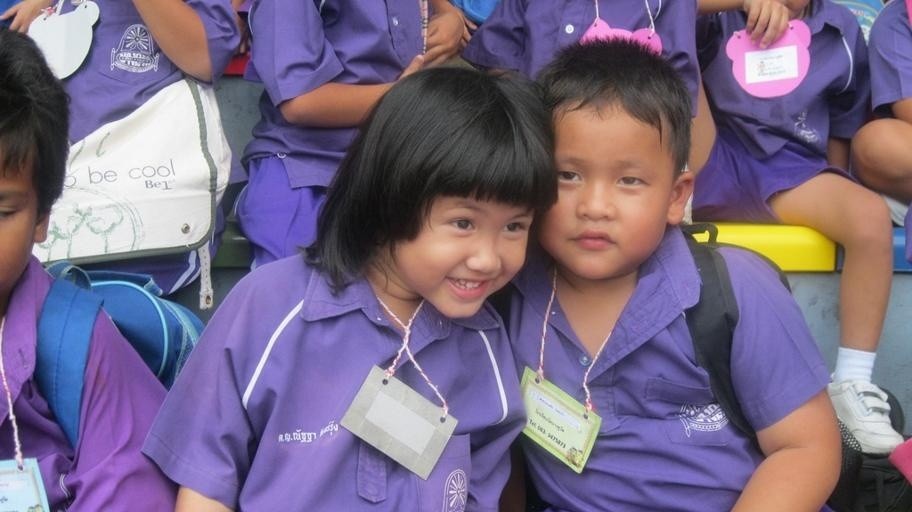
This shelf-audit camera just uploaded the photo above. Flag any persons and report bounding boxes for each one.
[235,0,465,266]
[465,2,719,225]
[137,64,556,511]
[1,0,241,294]
[0,27,179,512]
[849,0,912,201]
[694,0,908,458]
[428,0,499,48]
[498,35,844,512]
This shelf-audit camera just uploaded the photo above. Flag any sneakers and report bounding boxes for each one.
[827,372,904,454]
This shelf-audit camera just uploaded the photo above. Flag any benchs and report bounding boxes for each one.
[680,213,910,275]
[205,178,265,269]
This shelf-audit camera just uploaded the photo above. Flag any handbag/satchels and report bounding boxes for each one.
[32,76,232,266]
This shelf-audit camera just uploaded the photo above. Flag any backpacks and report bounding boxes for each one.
[35,262,205,450]
